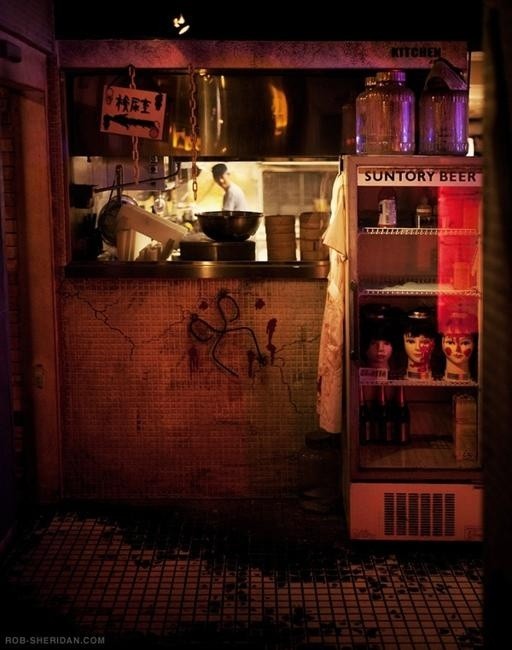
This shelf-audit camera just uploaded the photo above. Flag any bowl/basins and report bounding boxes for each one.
[267,211,332,262]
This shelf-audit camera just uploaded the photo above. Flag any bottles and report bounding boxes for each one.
[355,70,469,156]
[413,194,433,227]
[356,382,410,447]
[375,189,398,228]
[296,432,336,501]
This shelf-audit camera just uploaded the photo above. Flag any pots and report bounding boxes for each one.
[111,163,138,207]
[194,210,262,242]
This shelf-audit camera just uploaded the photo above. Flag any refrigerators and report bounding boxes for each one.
[342,154,486,545]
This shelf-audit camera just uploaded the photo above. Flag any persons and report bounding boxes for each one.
[212,165,248,212]
[439,315,477,382]
[401,309,437,380]
[360,330,394,374]
